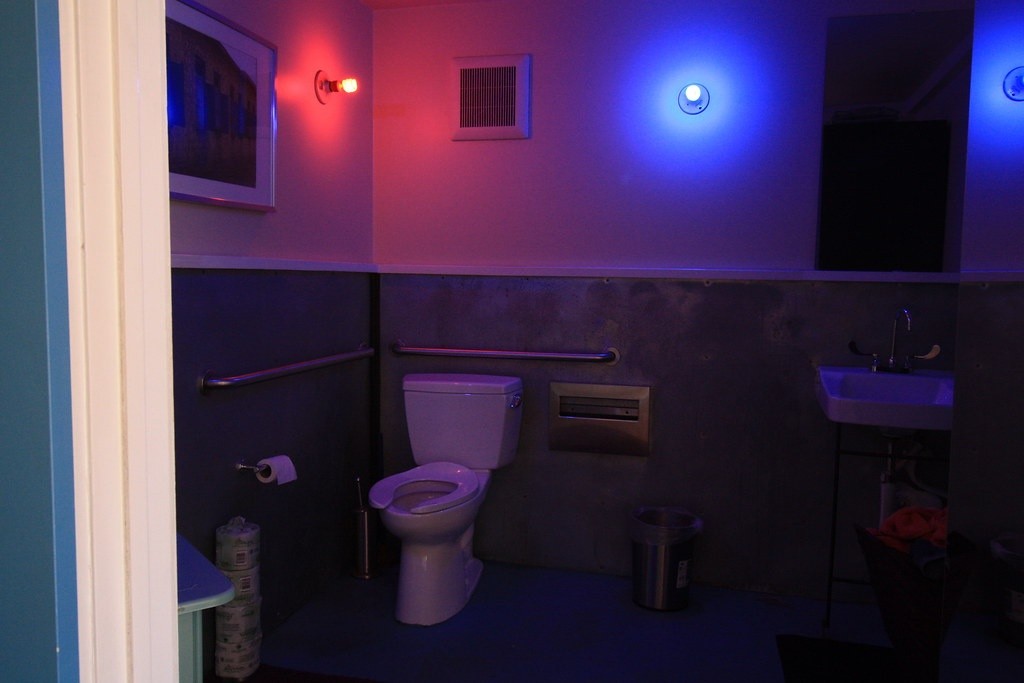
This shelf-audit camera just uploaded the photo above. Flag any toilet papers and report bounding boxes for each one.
[215,516,262,678]
[255,455,297,485]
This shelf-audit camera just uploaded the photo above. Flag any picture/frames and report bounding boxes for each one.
[165,0,278,211]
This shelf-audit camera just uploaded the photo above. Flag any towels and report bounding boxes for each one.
[862,506,948,556]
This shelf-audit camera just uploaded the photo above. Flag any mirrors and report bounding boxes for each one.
[814,9,975,275]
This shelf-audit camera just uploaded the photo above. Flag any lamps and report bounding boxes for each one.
[315,70,357,105]
[678,83,710,115]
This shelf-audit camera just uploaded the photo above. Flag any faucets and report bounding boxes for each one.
[888,309,911,368]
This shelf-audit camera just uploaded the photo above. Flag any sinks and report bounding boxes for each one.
[814,366,953,432]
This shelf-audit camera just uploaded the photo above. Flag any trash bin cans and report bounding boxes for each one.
[630,506,704,611]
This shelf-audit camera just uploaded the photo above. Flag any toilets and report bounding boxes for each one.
[367,373,524,626]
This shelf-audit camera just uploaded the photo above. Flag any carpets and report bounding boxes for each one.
[203,663,386,683]
[774,633,908,683]
